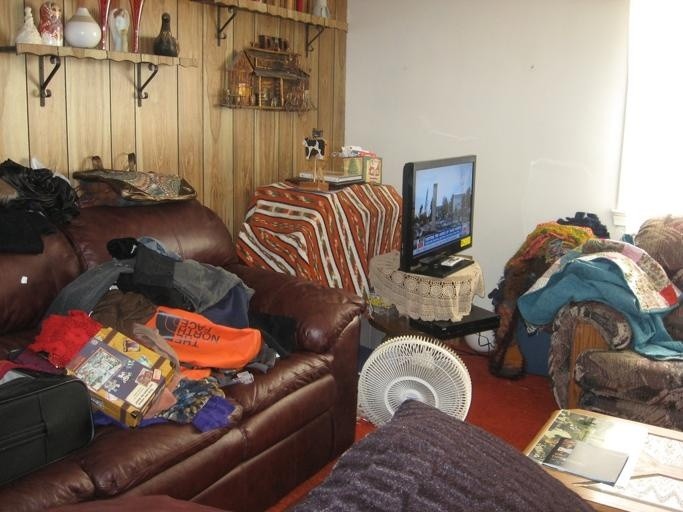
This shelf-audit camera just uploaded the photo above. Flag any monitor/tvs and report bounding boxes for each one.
[398,155,478,279]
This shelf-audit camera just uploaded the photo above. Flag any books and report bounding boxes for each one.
[299,170,363,183]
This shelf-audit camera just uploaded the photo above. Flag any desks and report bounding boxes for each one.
[520,407,683,512]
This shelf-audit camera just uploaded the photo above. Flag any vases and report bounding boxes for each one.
[62,0,102,49]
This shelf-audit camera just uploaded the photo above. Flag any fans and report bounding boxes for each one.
[356,334,472,432]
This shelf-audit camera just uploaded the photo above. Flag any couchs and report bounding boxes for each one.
[545,214,683,436]
[1,170,366,512]
